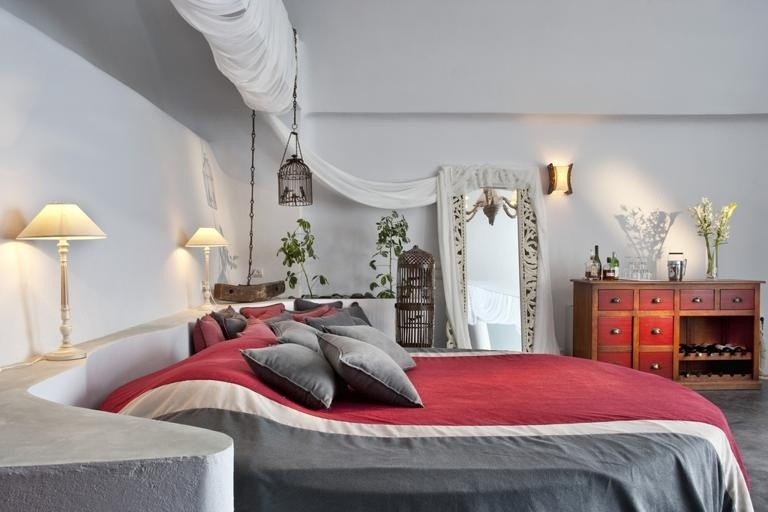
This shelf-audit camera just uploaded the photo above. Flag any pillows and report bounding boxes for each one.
[193,298,286,352]
[268,319,322,356]
[316,323,417,371]
[236,343,339,411]
[312,330,425,409]
[282,296,373,331]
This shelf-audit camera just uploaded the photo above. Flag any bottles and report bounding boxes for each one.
[584,254,594,279]
[611,251,619,280]
[593,245,602,281]
[602,257,615,280]
[681,341,747,358]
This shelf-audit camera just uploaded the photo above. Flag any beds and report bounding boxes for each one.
[101,347,755,512]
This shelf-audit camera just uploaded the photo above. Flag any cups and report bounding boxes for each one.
[590,262,600,280]
[667,252,686,280]
[623,257,655,280]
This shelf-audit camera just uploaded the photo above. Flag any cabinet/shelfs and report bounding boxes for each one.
[570,277,766,391]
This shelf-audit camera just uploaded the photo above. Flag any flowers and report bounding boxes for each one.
[691,195,739,277]
[616,206,681,263]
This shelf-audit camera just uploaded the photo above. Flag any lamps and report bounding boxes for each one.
[186,227,230,312]
[16,203,109,361]
[466,187,520,226]
[546,163,574,194]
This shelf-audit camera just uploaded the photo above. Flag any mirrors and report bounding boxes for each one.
[442,164,539,352]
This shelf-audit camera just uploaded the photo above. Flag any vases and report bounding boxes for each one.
[705,239,720,278]
